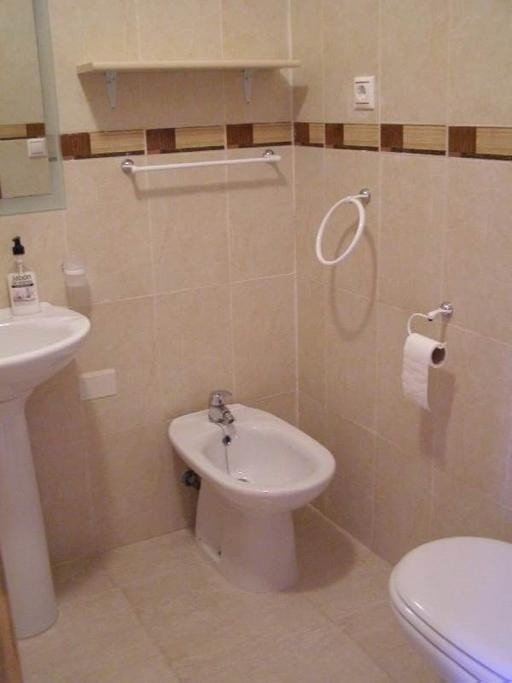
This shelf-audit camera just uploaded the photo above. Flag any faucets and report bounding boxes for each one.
[207,390,235,426]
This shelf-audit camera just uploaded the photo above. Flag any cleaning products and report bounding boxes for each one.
[7,237,40,314]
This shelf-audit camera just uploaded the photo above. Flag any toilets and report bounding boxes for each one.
[388,535,512,683]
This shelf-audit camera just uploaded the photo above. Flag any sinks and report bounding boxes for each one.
[0,302,91,402]
[167,402,337,594]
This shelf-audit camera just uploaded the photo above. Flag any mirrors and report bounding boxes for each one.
[0,0,67,216]
